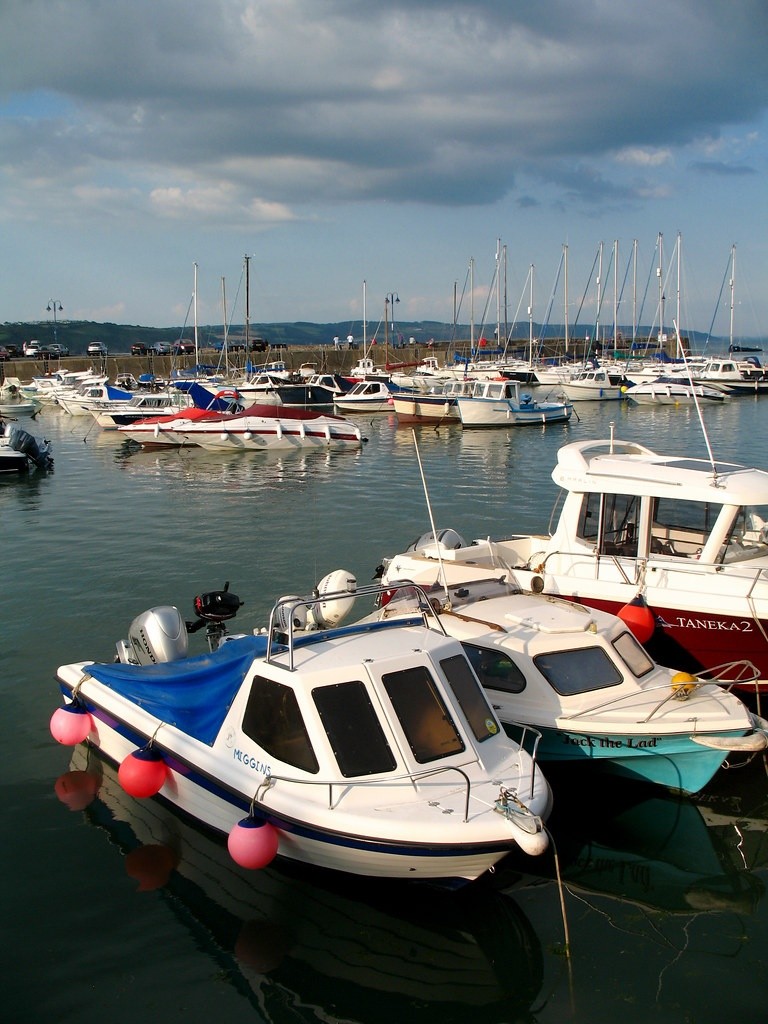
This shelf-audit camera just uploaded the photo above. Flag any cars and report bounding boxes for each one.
[131,341,150,355]
[87,342,108,356]
[150,341,172,355]
[169,339,196,354]
[0,344,47,358]
[47,343,69,356]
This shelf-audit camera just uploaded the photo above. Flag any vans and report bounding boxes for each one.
[31,340,41,346]
[248,337,266,352]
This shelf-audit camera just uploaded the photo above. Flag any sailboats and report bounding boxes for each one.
[333,231,768,429]
[174,253,299,408]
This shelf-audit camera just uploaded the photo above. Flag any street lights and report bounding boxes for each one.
[46,298,63,343]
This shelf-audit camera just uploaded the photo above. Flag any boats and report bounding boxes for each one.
[52,319,766,892]
[300,361,355,395]
[63,740,544,1023]
[1,352,193,473]
[514,761,768,914]
[117,390,364,452]
